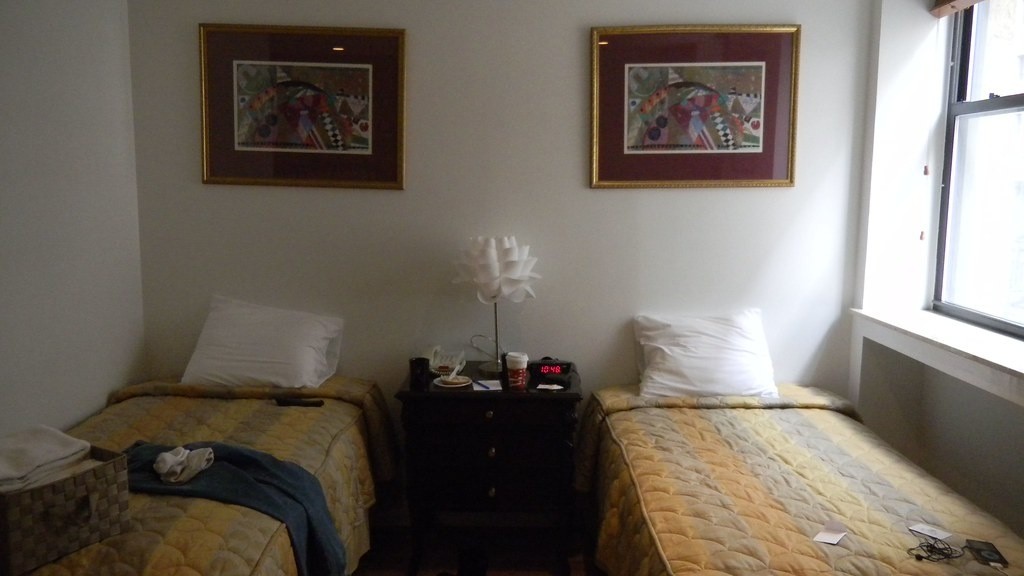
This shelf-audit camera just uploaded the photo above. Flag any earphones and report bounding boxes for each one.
[914,553,927,561]
[926,543,938,553]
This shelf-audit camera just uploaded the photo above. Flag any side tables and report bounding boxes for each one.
[392,353,582,576]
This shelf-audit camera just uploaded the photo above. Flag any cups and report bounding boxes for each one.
[506,352,528,390]
[409,357,429,376]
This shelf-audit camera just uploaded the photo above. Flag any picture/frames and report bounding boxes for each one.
[199,20,408,192]
[589,23,801,190]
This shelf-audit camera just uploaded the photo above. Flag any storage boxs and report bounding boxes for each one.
[0,445,131,576]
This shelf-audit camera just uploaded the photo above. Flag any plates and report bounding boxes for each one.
[434,377,473,387]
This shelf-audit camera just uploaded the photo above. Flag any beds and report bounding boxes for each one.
[1,366,399,576]
[586,382,1023,576]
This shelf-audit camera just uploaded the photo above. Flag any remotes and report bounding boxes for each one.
[274,397,325,407]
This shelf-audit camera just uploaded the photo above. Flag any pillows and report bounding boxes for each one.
[182,294,346,392]
[631,304,780,402]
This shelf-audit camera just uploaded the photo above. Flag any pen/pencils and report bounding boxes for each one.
[472,379,489,390]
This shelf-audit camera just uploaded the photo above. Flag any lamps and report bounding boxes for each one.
[445,231,538,374]
